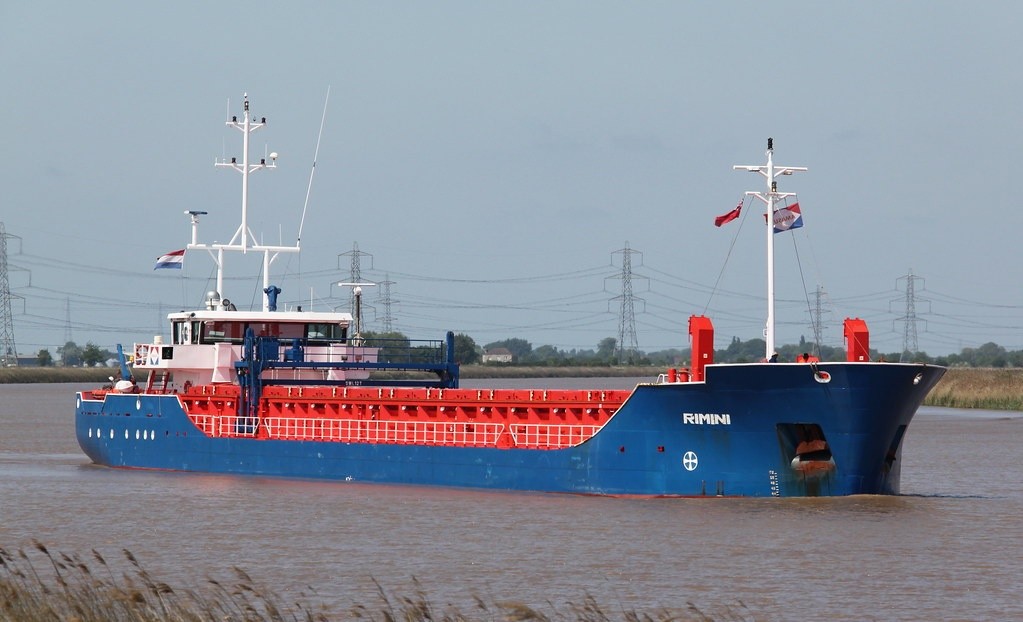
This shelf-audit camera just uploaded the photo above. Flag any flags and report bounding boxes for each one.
[763,202,803,234]
[153,249,185,271]
[715,197,744,227]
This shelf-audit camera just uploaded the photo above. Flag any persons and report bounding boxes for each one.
[769,352,778,363]
[799,353,809,363]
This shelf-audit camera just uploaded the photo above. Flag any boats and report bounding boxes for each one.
[75,90,950,500]
